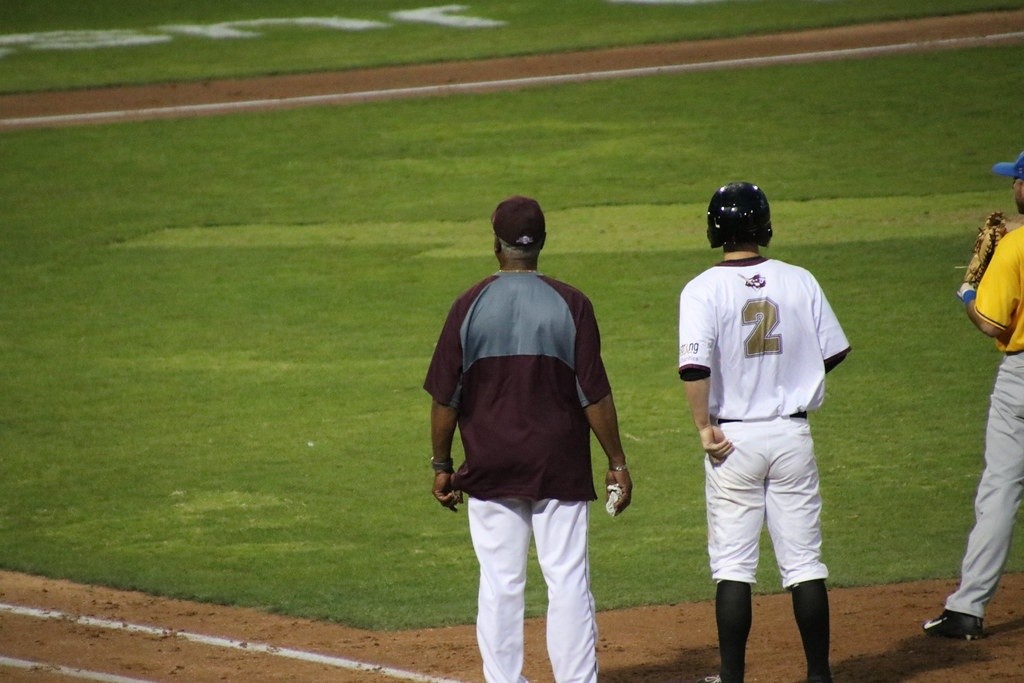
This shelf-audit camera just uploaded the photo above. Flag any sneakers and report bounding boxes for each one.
[922,609,983,641]
[697,675,722,683]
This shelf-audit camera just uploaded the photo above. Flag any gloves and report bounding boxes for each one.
[956,282,976,302]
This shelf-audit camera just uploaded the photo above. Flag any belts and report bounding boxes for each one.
[718,411,807,424]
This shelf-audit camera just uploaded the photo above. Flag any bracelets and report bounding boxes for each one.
[430,456,453,472]
[608,464,627,471]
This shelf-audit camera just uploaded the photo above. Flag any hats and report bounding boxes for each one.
[490,196,545,246]
[992,152,1024,179]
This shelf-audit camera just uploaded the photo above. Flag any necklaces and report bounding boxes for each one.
[499,268,540,273]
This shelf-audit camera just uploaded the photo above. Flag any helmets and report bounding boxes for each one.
[706,182,772,249]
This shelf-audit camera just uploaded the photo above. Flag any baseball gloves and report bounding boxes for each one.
[965,209,1007,286]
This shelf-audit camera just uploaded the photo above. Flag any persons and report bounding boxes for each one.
[924,150,1024,641]
[420,194,633,682]
[678,180,852,683]
